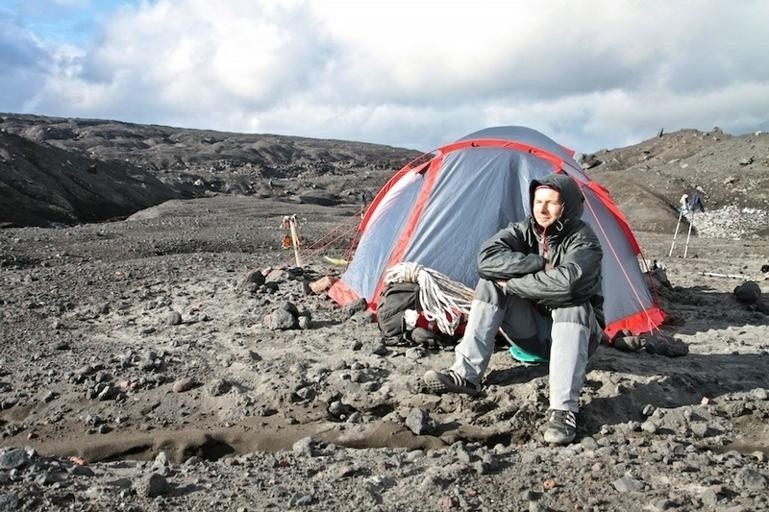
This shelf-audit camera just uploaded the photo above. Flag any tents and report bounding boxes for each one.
[324,125,667,341]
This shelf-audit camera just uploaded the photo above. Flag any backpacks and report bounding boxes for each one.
[376,281,419,346]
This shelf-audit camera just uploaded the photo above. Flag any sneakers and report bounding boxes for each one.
[544,408,576,443]
[424,369,482,395]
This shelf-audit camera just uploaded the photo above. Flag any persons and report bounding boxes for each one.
[423,173,607,445]
